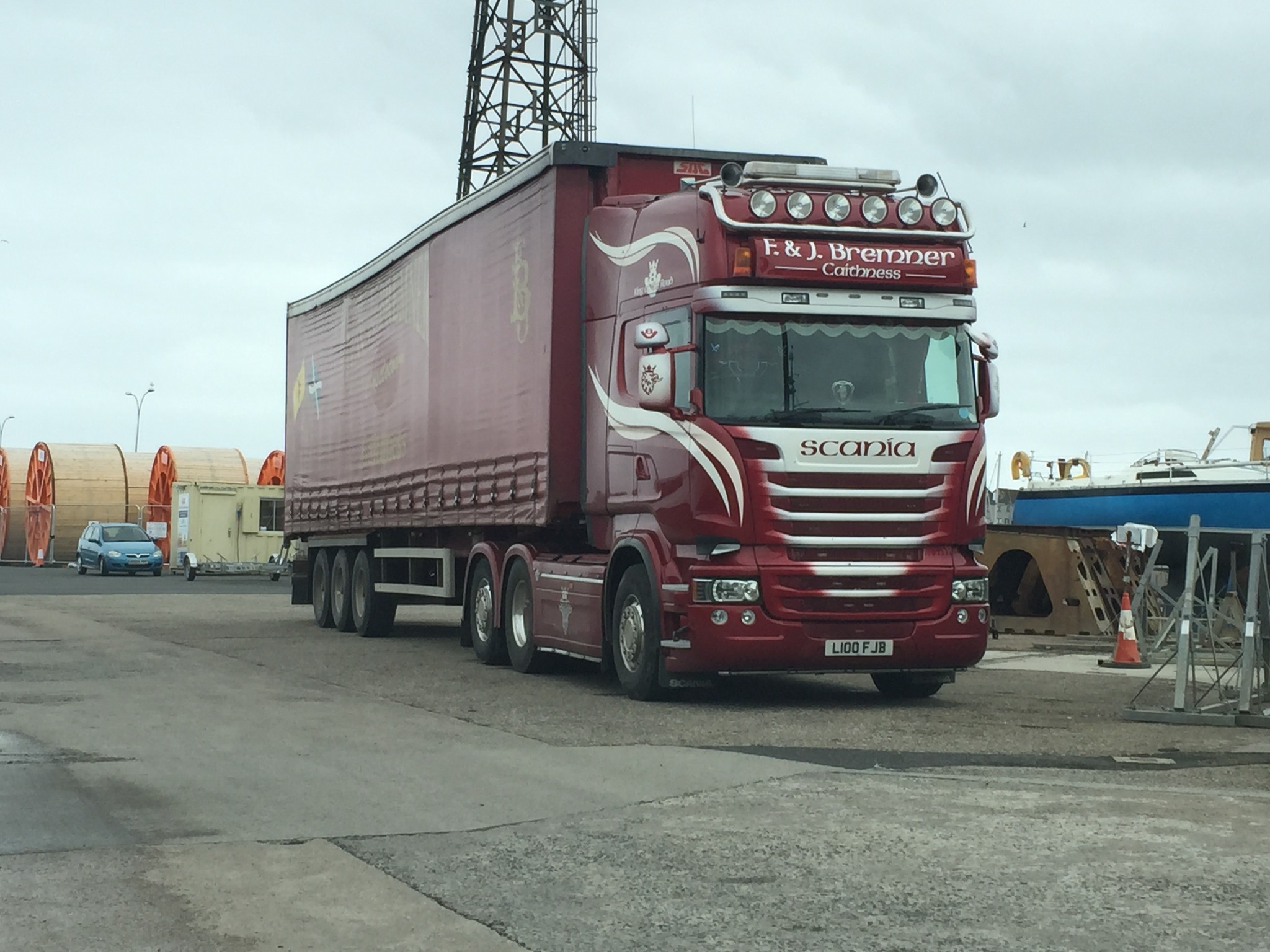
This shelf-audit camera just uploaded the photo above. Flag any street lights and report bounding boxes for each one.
[0,416,16,450]
[126,388,156,451]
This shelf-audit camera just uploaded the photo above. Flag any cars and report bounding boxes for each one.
[76,521,163,576]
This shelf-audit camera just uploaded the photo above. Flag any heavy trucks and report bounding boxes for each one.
[286,139,1004,700]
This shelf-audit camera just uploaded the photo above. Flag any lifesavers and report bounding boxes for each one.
[1012,451,1030,480]
[1064,457,1090,479]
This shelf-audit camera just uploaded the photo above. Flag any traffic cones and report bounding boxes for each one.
[1100,592,1151,670]
